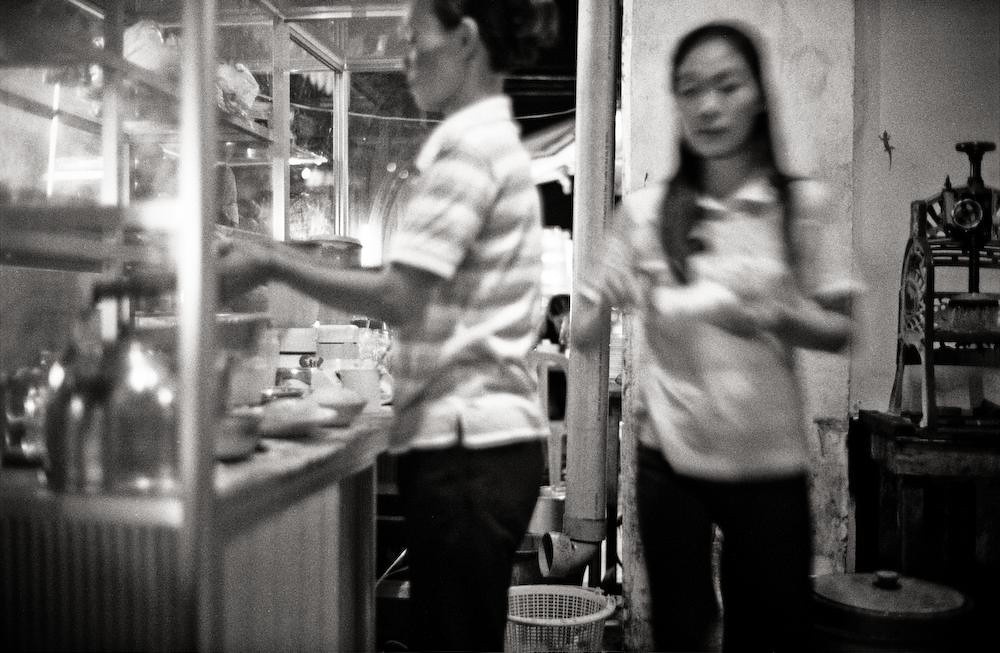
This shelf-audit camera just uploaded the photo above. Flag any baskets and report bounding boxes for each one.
[504,584,615,653]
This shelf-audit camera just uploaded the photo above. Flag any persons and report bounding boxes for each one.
[214,1,559,653]
[531,293,574,424]
[568,19,868,652]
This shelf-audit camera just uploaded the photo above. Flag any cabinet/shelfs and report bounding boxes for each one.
[889,186,1000,428]
[0,0,352,250]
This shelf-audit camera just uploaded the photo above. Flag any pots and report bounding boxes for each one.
[129,310,279,408]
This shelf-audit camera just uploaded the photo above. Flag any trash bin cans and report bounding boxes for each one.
[505,584,615,653]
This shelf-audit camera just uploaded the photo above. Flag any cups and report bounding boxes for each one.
[339,366,394,413]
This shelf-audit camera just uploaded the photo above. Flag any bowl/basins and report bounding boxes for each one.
[314,398,367,427]
[260,406,338,437]
[213,406,268,461]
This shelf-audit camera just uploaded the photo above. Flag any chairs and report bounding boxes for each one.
[531,353,569,486]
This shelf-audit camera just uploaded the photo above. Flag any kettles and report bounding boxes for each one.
[41,269,179,497]
